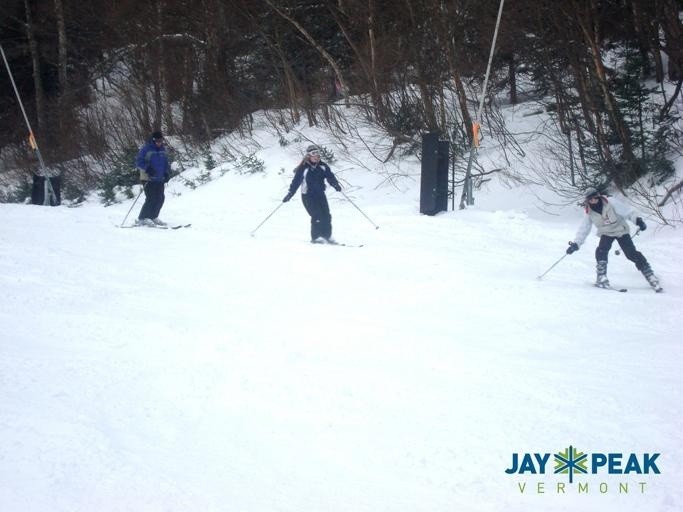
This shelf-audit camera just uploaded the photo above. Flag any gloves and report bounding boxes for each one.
[567,243,578,255]
[164,173,170,183]
[331,181,341,192]
[146,166,156,176]
[637,218,646,230]
[283,191,293,202]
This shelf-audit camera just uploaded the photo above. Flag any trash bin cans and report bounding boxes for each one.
[30,174,62,206]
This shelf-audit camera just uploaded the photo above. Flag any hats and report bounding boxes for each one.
[585,187,599,200]
[307,145,319,155]
[151,130,163,143]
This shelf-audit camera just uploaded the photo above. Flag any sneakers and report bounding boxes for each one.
[138,217,167,227]
[312,236,335,244]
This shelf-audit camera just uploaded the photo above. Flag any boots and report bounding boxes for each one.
[641,266,659,286]
[597,261,609,285]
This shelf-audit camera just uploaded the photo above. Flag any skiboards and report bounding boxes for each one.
[114,223,192,230]
[593,277,663,292]
[311,239,363,247]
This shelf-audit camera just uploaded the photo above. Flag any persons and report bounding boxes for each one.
[566,187,659,288]
[134,132,172,226]
[283,145,342,244]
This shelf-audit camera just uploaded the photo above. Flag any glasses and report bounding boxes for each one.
[306,149,320,156]
